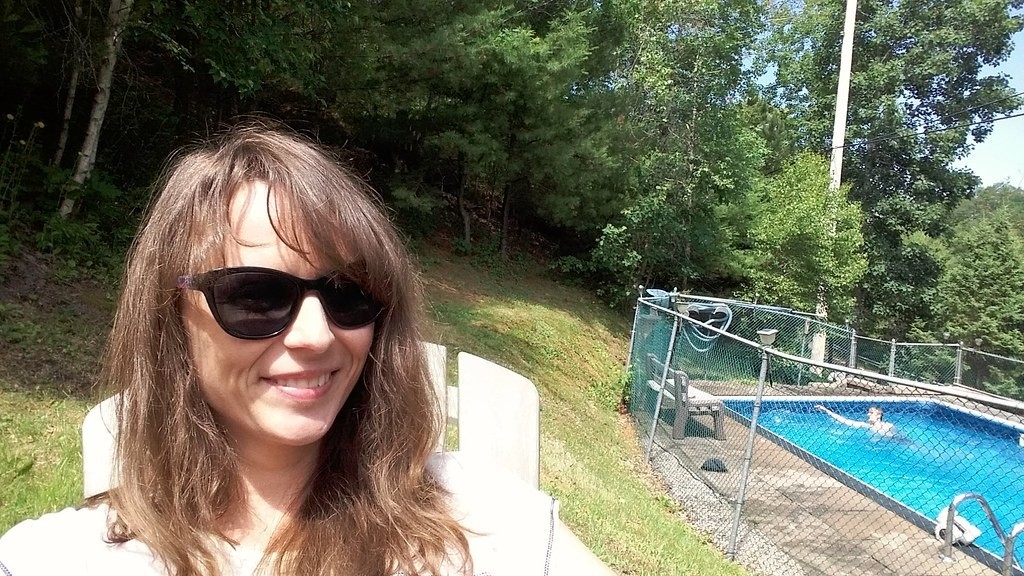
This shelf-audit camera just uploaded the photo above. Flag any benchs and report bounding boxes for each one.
[640,354,727,441]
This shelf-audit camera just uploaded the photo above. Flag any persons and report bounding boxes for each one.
[814,405,894,438]
[0,124,616,576]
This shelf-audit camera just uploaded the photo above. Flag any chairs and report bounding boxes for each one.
[83,338,539,576]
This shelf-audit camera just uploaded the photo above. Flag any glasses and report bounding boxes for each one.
[176,258,387,340]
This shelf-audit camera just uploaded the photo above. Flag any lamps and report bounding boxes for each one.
[676,298,689,314]
[756,328,778,346]
